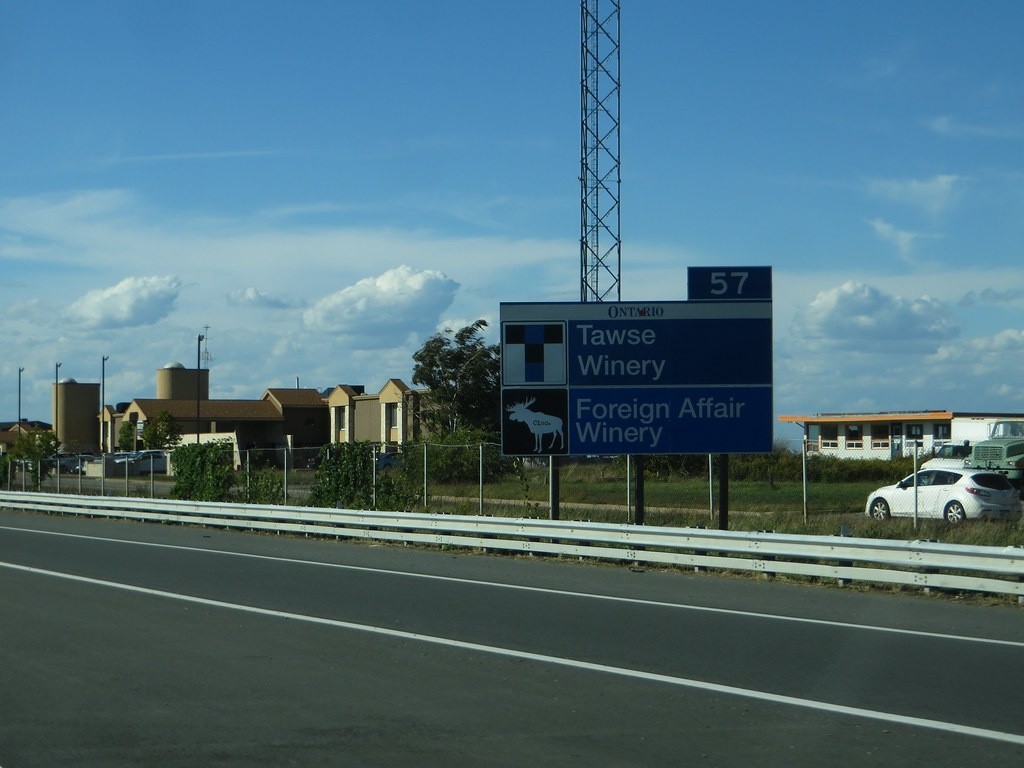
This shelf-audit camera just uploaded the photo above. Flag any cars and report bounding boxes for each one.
[865,468,1023,526]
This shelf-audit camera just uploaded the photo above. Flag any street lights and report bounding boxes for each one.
[18,366,26,433]
[102,355,110,453]
[55,362,62,455]
[196,332,205,444]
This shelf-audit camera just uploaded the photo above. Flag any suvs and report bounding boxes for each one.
[17,449,168,475]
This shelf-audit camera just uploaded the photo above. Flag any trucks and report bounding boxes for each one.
[919,416,1011,484]
[964,419,1024,501]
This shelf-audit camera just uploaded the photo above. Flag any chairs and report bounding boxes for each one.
[931,474,948,484]
